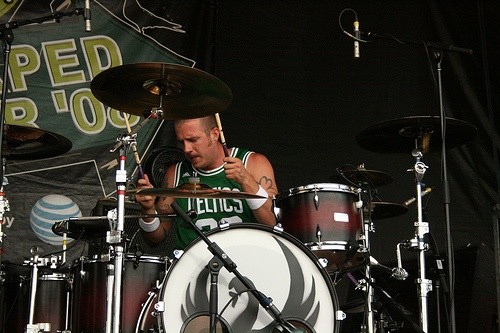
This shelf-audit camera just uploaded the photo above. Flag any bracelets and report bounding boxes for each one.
[138,210,161,232]
[246,185,269,209]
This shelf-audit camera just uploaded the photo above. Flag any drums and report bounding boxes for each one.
[274,181,373,277]
[10,271,77,333]
[71,253,173,333]
[136,221,341,333]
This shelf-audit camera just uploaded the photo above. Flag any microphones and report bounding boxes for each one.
[85,0,92,31]
[354,21,360,59]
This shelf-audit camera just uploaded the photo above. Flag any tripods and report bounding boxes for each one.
[403,185,436,206]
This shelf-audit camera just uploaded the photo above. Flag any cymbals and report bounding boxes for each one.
[89,61,233,121]
[332,167,396,193]
[136,183,267,201]
[360,200,410,221]
[0,123,72,162]
[355,115,481,157]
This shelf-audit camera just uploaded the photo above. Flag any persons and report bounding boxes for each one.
[135,115,280,257]
